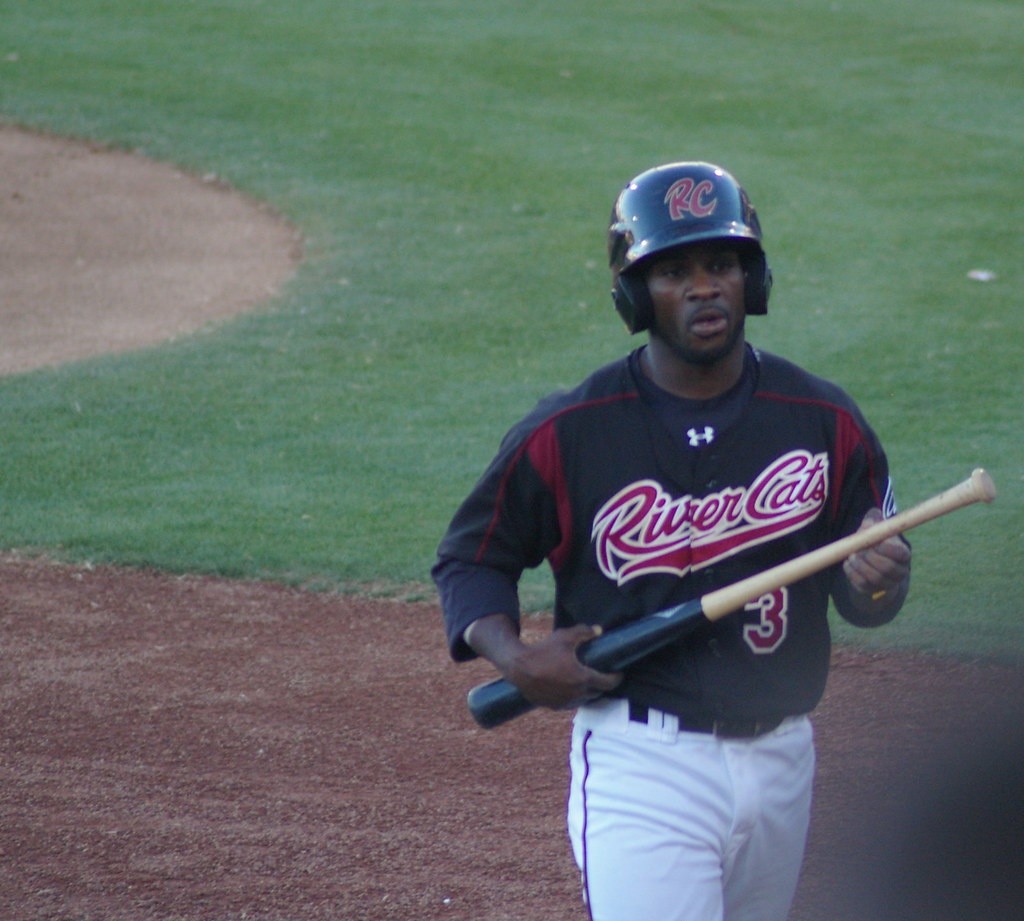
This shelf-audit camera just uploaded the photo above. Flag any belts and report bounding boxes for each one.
[628,699,786,738]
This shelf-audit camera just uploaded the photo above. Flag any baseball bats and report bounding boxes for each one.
[463,465,997,733]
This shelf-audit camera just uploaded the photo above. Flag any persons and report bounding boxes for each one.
[432,162,911,921]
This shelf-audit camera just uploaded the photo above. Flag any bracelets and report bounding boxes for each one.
[871,590,887,600]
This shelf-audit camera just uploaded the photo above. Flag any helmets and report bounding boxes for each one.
[606,162,772,334]
